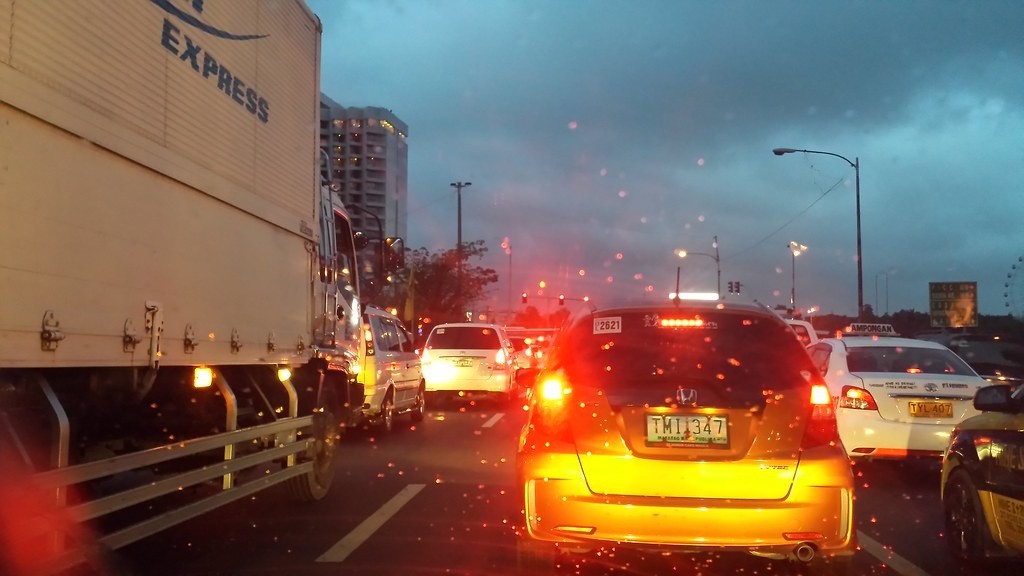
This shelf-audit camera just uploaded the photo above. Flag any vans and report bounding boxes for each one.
[362,307,426,431]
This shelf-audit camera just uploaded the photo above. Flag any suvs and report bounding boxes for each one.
[419,323,515,410]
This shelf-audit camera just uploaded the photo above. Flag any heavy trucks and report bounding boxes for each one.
[0,0,365,576]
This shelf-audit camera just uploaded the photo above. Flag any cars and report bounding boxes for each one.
[939,381,1024,567]
[512,301,858,557]
[801,322,989,457]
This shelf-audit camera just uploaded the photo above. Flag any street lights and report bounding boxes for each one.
[679,236,720,299]
[772,147,864,322]
[787,241,808,311]
[450,181,472,321]
[502,239,512,315]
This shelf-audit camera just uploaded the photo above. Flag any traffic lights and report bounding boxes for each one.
[728,281,733,293]
[734,281,740,293]
